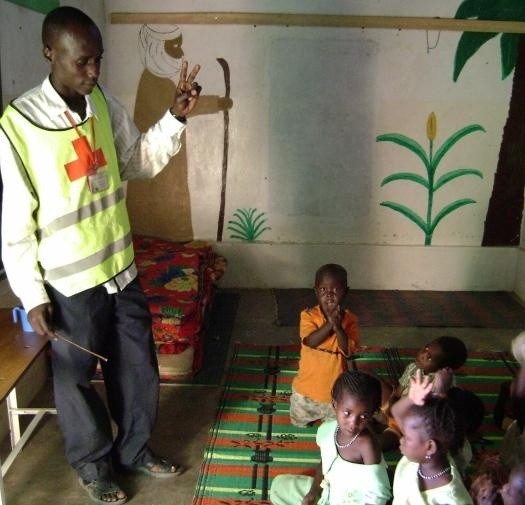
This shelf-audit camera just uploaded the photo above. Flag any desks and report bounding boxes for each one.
[0,307,63,505]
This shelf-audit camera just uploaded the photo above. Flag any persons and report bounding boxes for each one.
[289,264,360,429]
[125,22,233,242]
[269,335,525,504]
[0,7,203,505]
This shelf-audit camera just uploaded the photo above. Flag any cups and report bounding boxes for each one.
[12,307,35,333]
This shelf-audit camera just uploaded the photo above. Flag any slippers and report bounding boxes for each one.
[133,458,185,479]
[78,475,127,505]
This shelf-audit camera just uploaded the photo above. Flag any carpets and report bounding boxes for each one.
[268,288,525,329]
[190,338,523,504]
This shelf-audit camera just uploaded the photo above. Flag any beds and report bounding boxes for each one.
[0,233,229,382]
